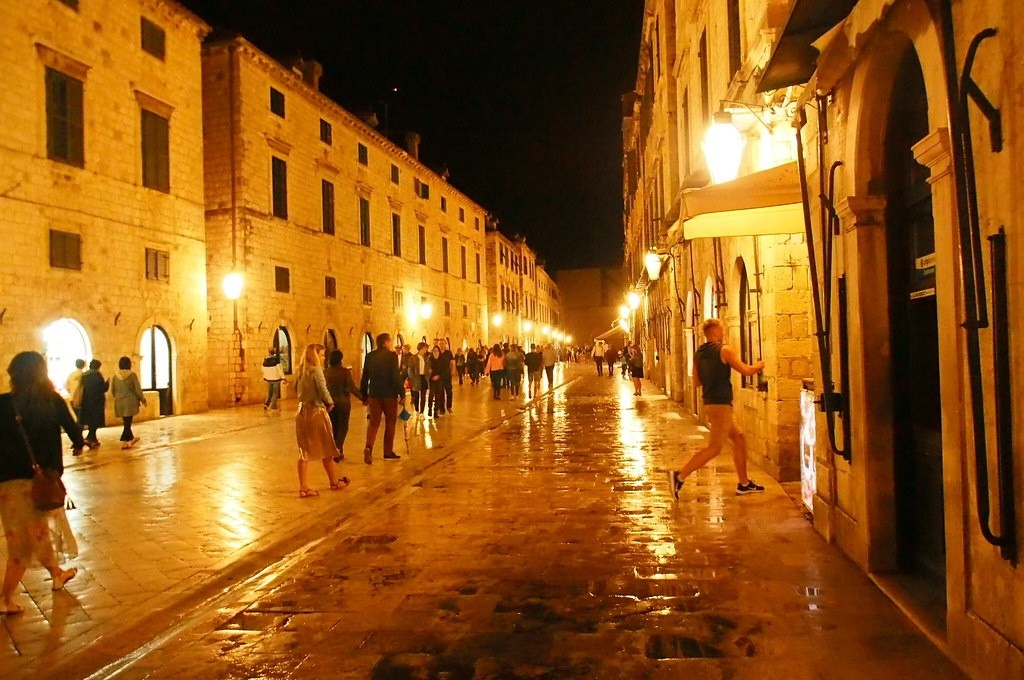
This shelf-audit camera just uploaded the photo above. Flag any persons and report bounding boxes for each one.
[394,338,456,420]
[669,318,766,501]
[605,344,616,376]
[358,333,406,464]
[621,340,632,375]
[455,343,557,401]
[323,349,368,463]
[0,351,85,613]
[295,343,351,498]
[629,343,644,396]
[111,356,147,449]
[262,347,287,411]
[590,342,604,376]
[566,344,586,363]
[77,359,110,449]
[65,359,86,407]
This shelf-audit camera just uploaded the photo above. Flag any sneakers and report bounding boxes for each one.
[670,470,685,501]
[364,445,373,465]
[383,452,401,461]
[736,479,766,494]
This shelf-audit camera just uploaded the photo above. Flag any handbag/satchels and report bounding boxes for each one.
[31,463,76,511]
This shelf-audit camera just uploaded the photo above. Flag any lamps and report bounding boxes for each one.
[702,99,774,185]
[645,243,669,280]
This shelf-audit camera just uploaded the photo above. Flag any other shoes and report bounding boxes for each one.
[131,437,141,445]
[515,394,519,400]
[510,396,514,400]
[121,444,132,450]
[447,408,453,414]
[420,414,426,420]
[428,409,432,416]
[440,410,445,415]
[529,391,532,399]
[534,395,537,398]
[415,411,420,418]
[92,441,101,446]
[593,371,644,397]
[83,439,95,449]
[434,414,439,418]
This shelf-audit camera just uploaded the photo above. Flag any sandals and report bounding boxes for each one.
[0,602,24,615]
[328,476,351,491]
[298,488,320,499]
[51,567,78,591]
